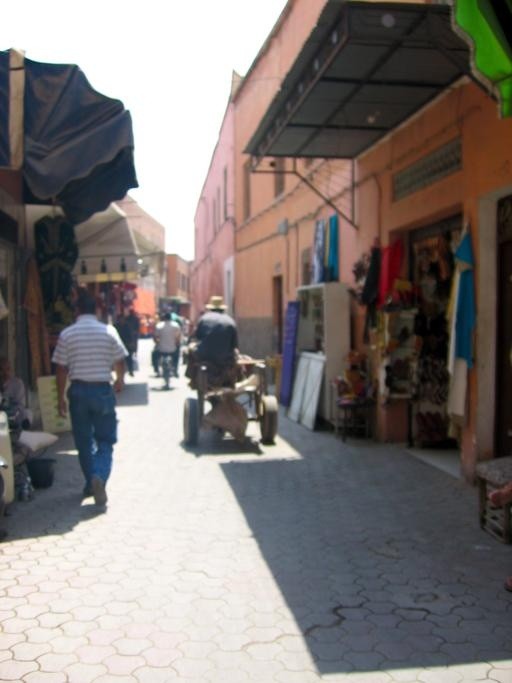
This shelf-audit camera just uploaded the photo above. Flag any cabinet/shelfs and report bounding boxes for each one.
[297,281,352,424]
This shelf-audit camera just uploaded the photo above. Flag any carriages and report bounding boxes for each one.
[184,348,278,444]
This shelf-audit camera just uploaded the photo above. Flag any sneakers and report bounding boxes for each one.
[81,473,106,505]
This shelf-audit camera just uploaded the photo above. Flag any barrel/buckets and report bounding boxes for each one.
[26,457,56,489]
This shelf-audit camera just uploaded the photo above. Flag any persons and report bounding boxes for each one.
[168,305,182,349]
[51,289,131,507]
[116,303,136,376]
[152,310,180,376]
[189,295,239,362]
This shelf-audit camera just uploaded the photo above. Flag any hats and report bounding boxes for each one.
[204,295,228,310]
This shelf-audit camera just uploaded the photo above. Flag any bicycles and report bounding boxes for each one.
[160,352,174,390]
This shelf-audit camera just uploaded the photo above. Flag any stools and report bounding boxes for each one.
[474,456,512,543]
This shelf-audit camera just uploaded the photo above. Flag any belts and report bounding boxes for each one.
[71,379,109,386]
